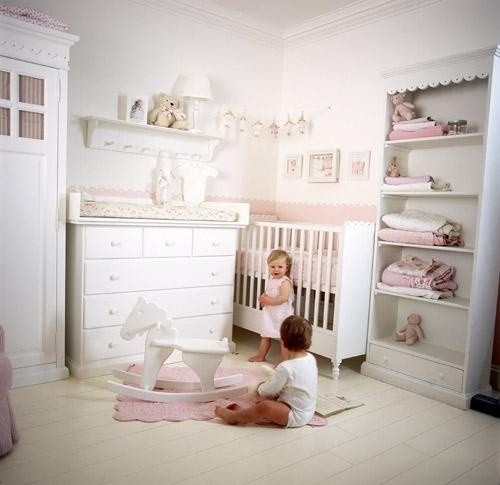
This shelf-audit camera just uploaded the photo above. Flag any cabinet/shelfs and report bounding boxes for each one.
[66,191,252,380]
[359,42,500,414]
[0,12,83,392]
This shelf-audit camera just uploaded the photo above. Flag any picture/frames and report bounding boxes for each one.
[305,148,340,184]
[345,148,373,184]
[282,153,304,180]
[126,92,149,125]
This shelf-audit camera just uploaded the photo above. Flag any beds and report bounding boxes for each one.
[232,212,371,381]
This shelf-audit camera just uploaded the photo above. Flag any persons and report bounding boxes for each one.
[247,250,295,362]
[214,314,319,427]
[131,99,144,116]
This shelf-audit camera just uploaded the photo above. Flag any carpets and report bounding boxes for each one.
[110,362,328,427]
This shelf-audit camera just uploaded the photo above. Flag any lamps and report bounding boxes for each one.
[174,70,213,132]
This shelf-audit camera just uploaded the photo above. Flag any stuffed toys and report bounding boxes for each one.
[390,92,416,122]
[390,313,424,345]
[149,93,189,130]
